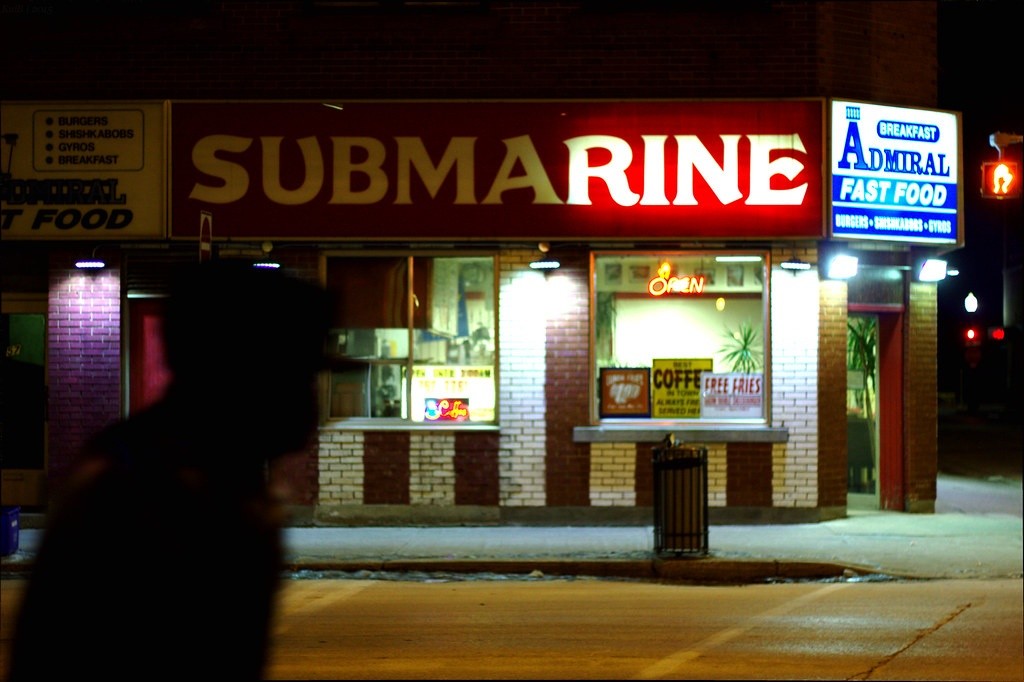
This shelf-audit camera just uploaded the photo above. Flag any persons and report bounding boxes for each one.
[3,256,367,682]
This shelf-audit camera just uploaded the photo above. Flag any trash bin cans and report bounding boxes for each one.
[649,446,709,558]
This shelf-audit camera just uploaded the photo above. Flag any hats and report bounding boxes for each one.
[166,257,370,372]
